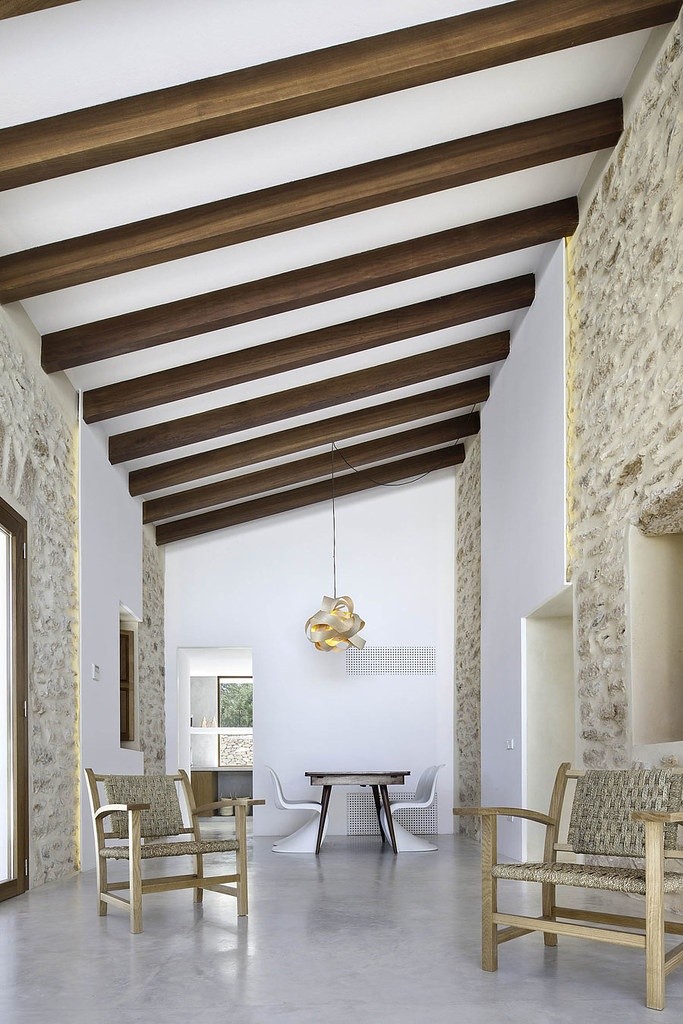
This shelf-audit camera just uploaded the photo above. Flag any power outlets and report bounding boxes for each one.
[507,739,513,750]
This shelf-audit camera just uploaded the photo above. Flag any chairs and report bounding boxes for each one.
[453,763,683,1011]
[380,763,445,852]
[265,765,329,853]
[84,767,265,934]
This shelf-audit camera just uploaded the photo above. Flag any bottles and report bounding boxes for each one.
[211,716,215,727]
[201,717,207,728]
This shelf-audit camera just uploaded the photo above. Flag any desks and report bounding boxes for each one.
[304,769,411,853]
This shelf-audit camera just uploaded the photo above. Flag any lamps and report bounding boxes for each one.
[304,442,367,652]
